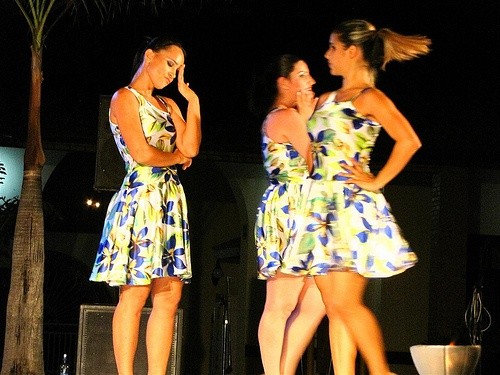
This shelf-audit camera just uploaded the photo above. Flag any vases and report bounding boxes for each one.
[409,345,482,375]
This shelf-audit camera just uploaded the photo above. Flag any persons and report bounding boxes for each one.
[298,18,432,375]
[254,49,327,375]
[88,35,203,375]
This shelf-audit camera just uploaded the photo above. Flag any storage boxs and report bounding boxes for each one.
[76,305,184,375]
